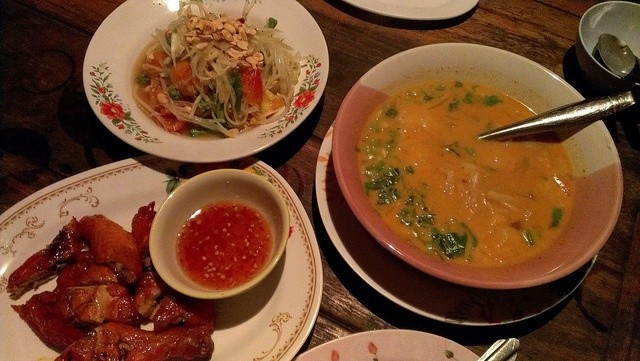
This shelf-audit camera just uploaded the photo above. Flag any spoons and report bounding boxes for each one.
[598,34,637,86]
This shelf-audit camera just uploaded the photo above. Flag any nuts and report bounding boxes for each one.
[188,15,262,67]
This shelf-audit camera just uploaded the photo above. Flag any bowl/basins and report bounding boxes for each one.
[148,168,291,299]
[332,42,624,291]
[576,1,637,84]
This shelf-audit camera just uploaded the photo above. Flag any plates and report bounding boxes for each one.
[81,0,330,163]
[1,154,324,361]
[341,0,480,24]
[293,329,482,360]
[314,118,597,329]
